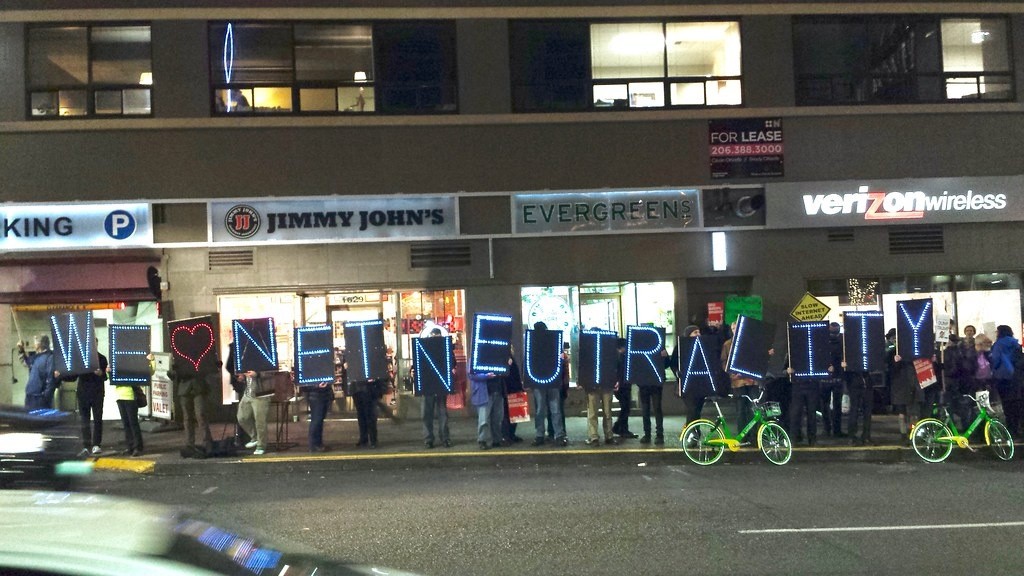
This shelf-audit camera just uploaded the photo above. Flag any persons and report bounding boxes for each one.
[291,366,335,453]
[106,353,157,457]
[225,342,276,454]
[17,334,56,408]
[721,316,1024,447]
[235,91,250,107]
[54,337,108,454]
[637,349,671,444]
[469,320,640,450]
[344,362,386,448]
[410,328,457,449]
[670,325,706,447]
[167,353,223,458]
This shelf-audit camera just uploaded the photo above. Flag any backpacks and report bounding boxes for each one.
[975,351,991,379]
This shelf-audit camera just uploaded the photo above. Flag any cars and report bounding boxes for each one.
[1,401,418,575]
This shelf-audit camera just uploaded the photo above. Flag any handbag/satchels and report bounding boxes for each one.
[445,391,465,409]
[993,346,1015,380]
[137,388,147,407]
[507,390,531,424]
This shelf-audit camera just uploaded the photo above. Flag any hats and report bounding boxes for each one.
[683,326,699,337]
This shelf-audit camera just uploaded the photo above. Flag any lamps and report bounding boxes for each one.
[140,72,153,85]
[354,72,366,80]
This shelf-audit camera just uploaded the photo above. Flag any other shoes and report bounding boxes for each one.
[309,444,330,452]
[607,438,618,445]
[246,441,258,448]
[479,434,569,450]
[591,439,600,447]
[443,439,453,447]
[640,435,650,444]
[425,442,433,448]
[82,448,90,455]
[121,449,132,455]
[655,437,663,445]
[132,449,140,456]
[356,442,377,449]
[91,445,102,454]
[254,449,265,455]
[612,428,639,438]
[790,430,914,449]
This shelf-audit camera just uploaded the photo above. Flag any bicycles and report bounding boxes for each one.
[680,385,793,467]
[908,391,1015,463]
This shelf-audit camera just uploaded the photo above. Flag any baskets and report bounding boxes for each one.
[975,403,1004,418]
[752,401,782,419]
